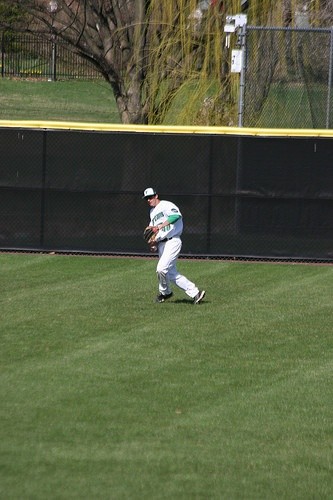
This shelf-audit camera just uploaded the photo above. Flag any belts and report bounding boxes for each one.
[159,237,172,242]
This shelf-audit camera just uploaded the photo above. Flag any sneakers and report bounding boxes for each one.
[157,292,172,303]
[193,291,205,304]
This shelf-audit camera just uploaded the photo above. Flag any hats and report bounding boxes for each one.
[141,188,156,199]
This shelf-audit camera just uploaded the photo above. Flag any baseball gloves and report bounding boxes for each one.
[143,226,159,244]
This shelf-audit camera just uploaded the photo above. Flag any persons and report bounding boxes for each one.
[141,187,206,304]
